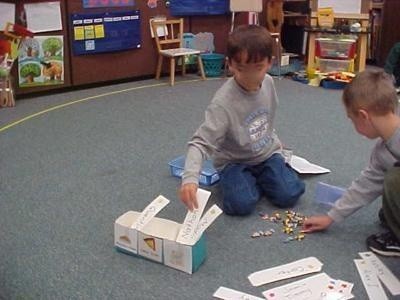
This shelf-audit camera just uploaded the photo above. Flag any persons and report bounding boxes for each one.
[179,24,305,216]
[303,67,400,258]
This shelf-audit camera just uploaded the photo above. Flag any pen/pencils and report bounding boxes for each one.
[299,230,307,234]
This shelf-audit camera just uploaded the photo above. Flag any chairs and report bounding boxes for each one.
[151,18,207,87]
[223,0,283,85]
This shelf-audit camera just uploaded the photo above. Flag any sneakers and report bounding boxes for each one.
[365,232,400,256]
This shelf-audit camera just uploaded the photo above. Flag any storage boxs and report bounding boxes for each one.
[315,33,358,74]
